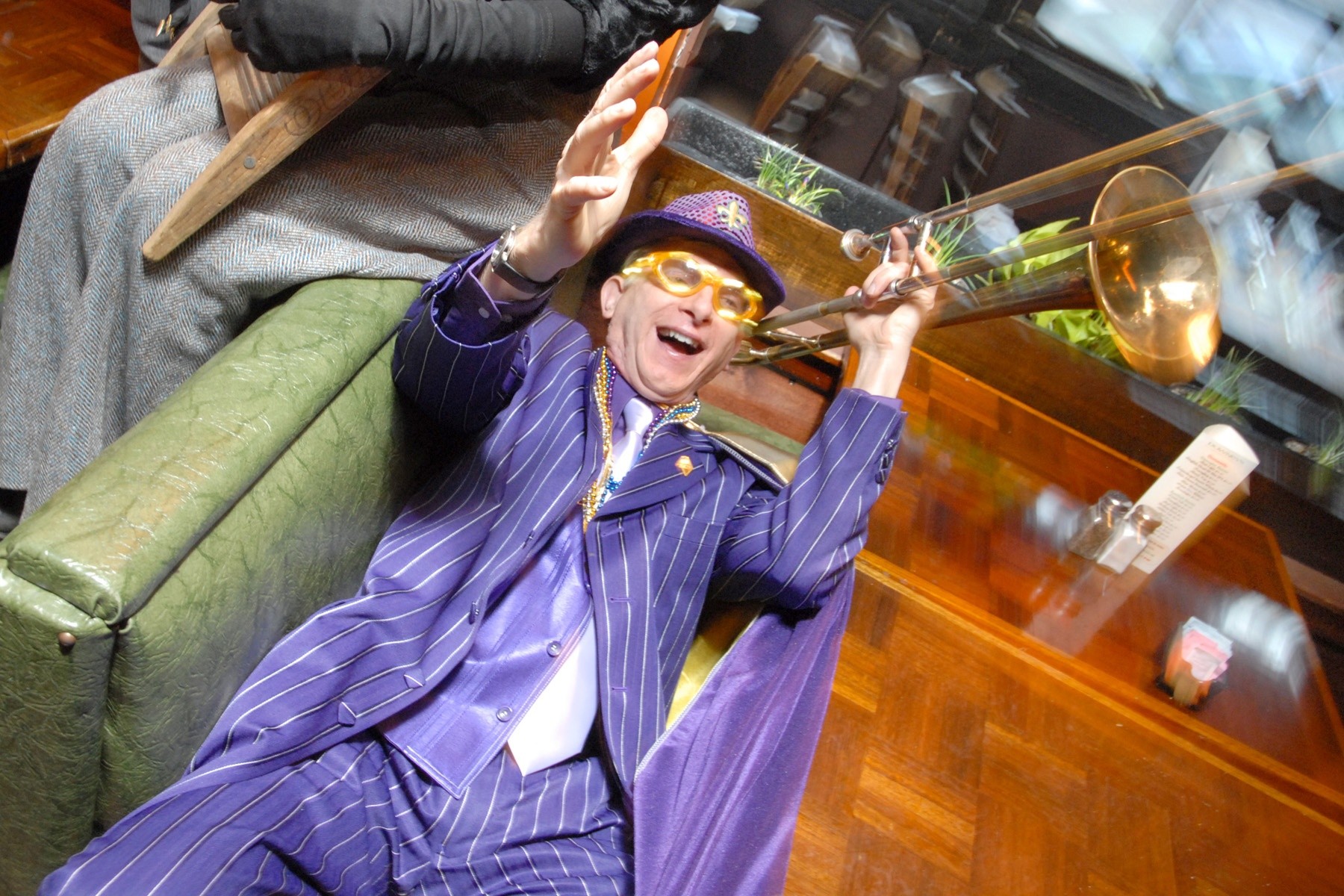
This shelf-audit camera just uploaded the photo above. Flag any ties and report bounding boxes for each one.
[509,395,654,777]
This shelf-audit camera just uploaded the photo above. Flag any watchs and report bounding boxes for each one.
[490,223,568,300]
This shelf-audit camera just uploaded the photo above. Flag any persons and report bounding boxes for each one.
[0,0,718,541]
[38,41,940,896]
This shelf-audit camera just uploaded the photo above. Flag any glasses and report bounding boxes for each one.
[622,251,763,337]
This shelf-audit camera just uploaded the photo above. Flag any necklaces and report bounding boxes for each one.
[584,343,702,544]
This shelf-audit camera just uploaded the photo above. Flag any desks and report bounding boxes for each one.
[787,344,1343,896]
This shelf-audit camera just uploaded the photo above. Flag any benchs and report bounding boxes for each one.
[1,278,809,896]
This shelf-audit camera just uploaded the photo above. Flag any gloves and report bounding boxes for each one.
[211,0,554,73]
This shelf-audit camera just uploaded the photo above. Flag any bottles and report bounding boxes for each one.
[1026,530,1119,619]
[1063,489,1163,576]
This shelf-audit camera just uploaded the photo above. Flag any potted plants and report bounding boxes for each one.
[648,95,1344,581]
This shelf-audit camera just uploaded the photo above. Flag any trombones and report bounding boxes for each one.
[723,61,1344,389]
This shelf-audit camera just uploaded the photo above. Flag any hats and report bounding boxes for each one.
[600,190,785,324]
